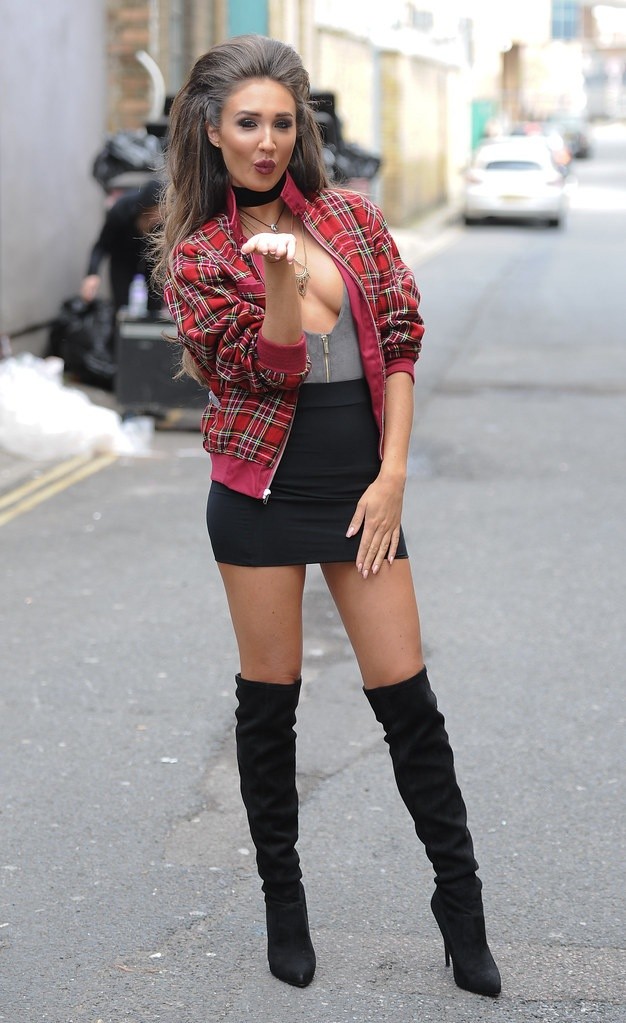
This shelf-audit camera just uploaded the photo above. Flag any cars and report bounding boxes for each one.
[508,120,587,174]
[464,139,563,230]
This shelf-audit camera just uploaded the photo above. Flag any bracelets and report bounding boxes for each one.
[263,257,287,265]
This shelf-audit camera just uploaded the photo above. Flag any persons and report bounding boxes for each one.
[148,33,507,1003]
[79,179,176,358]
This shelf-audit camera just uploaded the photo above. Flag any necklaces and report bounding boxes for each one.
[230,204,312,300]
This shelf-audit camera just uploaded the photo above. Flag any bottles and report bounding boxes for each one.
[129,275,148,320]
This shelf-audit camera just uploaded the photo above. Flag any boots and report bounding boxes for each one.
[234,674,316,987]
[362,665,503,997]
